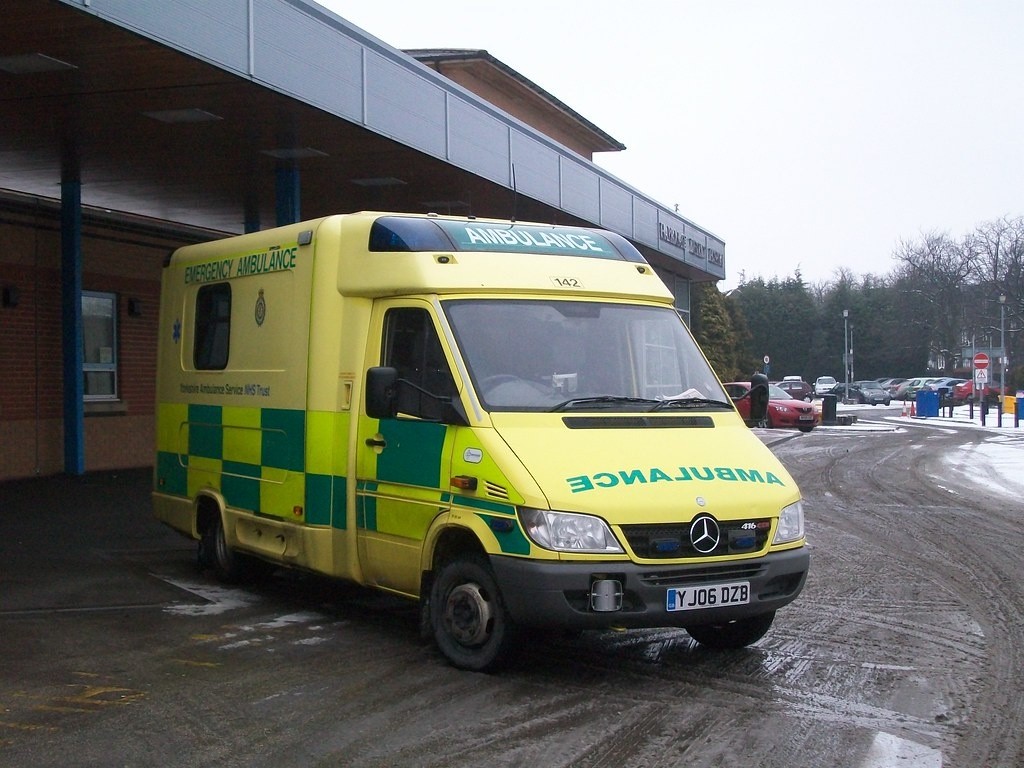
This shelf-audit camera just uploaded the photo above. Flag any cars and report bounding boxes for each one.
[930,379,968,394]
[723,382,820,433]
[774,382,814,403]
[854,381,891,406]
[953,378,1011,403]
[826,383,850,401]
[875,377,947,400]
[812,376,840,397]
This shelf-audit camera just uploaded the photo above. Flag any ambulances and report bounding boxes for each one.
[151,211,810,672]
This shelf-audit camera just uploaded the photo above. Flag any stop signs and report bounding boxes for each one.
[973,353,989,369]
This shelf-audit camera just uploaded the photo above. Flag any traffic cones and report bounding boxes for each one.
[909,401,916,416]
[900,401,908,416]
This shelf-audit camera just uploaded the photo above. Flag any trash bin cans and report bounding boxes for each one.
[916,390,940,417]
[821,394,837,421]
[1016,398,1024,419]
[983,395,989,414]
[998,395,1016,414]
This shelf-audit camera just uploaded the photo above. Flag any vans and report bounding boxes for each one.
[783,376,802,382]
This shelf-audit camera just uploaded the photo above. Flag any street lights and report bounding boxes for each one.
[999,293,1007,413]
[843,309,849,399]
[850,324,854,382]
